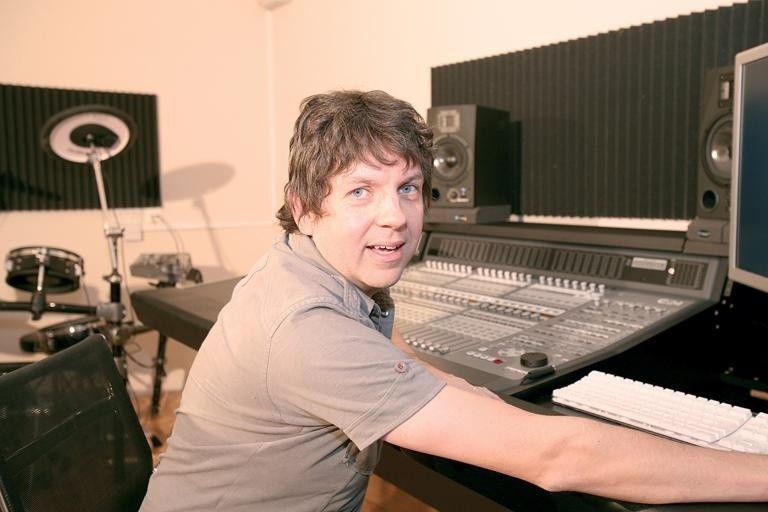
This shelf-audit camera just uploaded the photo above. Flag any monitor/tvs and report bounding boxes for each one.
[728,44,768,293]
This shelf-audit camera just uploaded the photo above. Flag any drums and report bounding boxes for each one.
[37,317,114,357]
[5,246,85,294]
[19,331,45,356]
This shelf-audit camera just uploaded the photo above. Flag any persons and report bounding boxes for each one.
[135,89,767,512]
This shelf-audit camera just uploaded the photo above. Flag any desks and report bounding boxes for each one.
[131,274,768,512]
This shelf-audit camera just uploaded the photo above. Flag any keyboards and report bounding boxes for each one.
[553,370,768,454]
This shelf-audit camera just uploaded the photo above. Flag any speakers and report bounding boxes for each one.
[697,64,735,220]
[428,104,509,208]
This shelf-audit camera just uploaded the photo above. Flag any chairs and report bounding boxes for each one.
[0,332,154,512]
